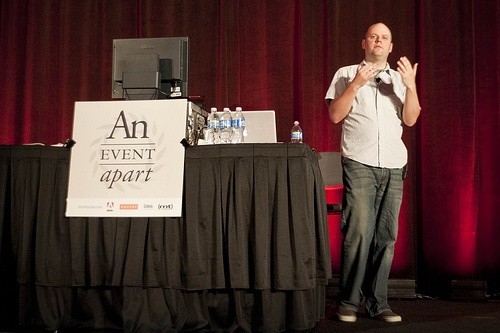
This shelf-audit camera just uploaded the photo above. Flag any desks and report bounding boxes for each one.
[0,143,332,333]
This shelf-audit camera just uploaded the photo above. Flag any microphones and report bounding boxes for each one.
[376,78,381,83]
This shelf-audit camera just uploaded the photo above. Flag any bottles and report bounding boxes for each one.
[207,108,219,144]
[232,107,246,144]
[219,108,232,144]
[291,121,303,144]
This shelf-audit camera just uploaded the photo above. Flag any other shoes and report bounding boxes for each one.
[336,305,358,322]
[373,309,402,322]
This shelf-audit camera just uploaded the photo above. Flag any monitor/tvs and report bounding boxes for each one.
[112,37,188,98]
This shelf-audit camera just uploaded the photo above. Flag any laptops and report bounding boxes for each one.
[216,110,284,143]
